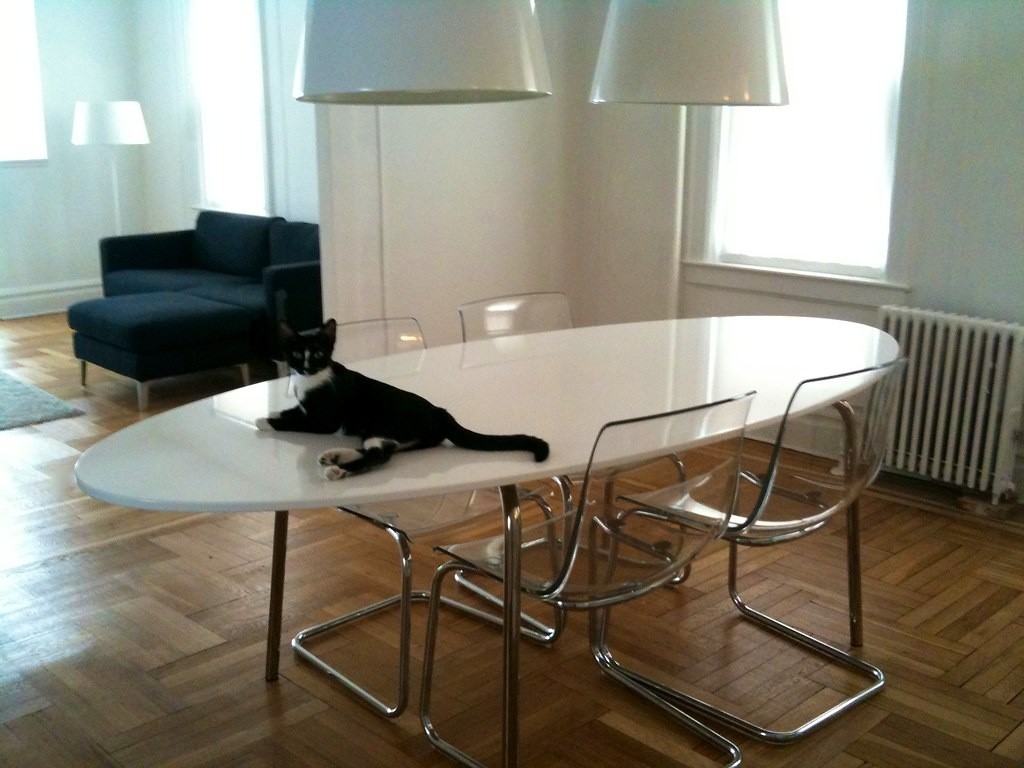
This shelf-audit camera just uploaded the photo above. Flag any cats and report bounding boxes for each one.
[255,319,549,480]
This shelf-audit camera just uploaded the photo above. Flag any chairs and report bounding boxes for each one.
[292,292,908,768]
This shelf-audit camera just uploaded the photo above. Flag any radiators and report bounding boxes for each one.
[856,301,1024,503]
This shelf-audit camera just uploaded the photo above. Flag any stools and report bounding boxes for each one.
[68,289,256,411]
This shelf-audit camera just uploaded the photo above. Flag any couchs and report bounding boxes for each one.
[99,210,320,364]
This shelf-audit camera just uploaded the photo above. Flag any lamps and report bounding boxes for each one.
[297,1,552,110]
[589,0,790,110]
[70,97,151,236]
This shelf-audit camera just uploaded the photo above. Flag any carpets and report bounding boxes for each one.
[0,371,84,434]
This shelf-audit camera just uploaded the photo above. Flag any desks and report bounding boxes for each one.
[73,313,901,768]
